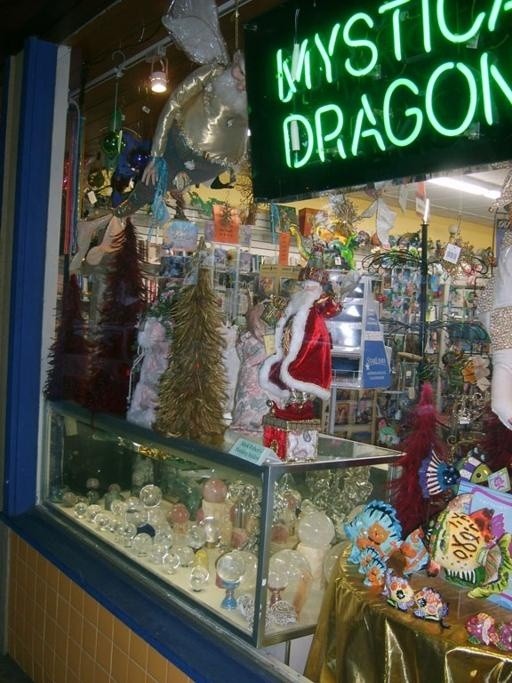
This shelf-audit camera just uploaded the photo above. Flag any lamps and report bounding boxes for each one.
[148,72,168,93]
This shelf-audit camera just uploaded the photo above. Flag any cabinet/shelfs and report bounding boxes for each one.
[40,397,407,649]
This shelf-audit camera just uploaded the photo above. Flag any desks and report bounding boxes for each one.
[303,525,512,683]
